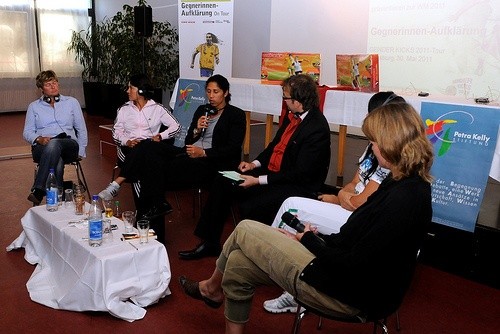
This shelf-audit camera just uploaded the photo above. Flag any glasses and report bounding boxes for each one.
[282,96,292,101]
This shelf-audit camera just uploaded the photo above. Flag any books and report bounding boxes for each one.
[121,228,158,243]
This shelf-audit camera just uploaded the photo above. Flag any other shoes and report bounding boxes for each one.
[27,189,43,205]
[179,277,223,309]
[164,201,174,214]
[178,243,218,260]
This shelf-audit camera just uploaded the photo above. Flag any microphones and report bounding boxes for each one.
[201,107,210,136]
[282,212,326,246]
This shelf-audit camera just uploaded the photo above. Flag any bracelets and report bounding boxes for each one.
[156,135,161,140]
[192,129,200,135]
[36,137,41,144]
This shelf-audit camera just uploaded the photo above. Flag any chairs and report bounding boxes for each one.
[33,158,91,200]
[292,231,432,334]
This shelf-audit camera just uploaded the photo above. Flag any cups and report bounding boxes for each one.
[65,189,75,206]
[58,187,63,206]
[104,199,114,217]
[75,184,86,214]
[122,211,135,232]
[101,219,112,239]
[137,220,150,243]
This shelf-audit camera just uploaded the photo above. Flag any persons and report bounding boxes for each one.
[286,54,361,89]
[180,101,433,334]
[132,74,247,243]
[263,91,408,314]
[97,72,183,210]
[178,73,332,260]
[21,70,88,206]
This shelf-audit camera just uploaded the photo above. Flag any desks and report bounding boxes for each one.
[31,201,162,308]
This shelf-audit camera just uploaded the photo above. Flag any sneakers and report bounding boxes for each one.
[263,291,307,313]
[98,180,121,200]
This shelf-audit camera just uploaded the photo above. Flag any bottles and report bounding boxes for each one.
[114,201,123,220]
[88,195,103,247]
[46,169,58,212]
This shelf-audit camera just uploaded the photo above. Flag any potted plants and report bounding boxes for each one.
[78,16,162,120]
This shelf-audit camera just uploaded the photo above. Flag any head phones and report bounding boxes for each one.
[208,102,229,114]
[138,77,148,94]
[294,109,309,119]
[44,94,60,103]
